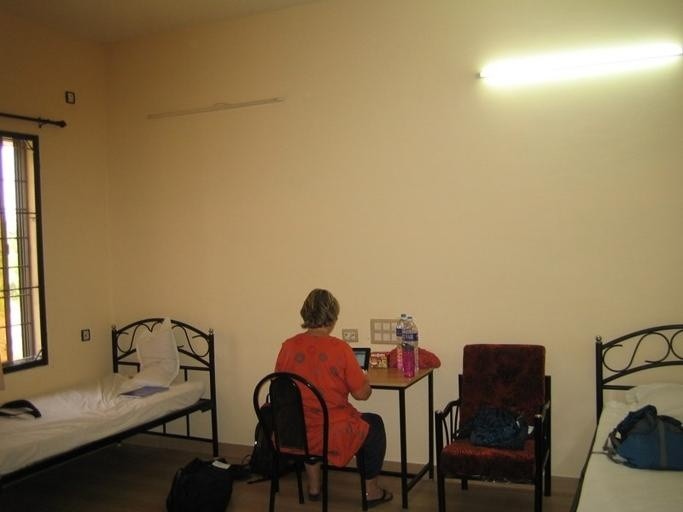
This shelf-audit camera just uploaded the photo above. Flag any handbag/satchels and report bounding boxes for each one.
[450,406,528,451]
[165,454,251,512]
[602,404,682,472]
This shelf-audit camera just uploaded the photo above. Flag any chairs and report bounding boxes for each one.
[251,372,368,510]
[432,344,551,511]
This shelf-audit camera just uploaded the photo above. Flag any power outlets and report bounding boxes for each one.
[342,329,359,344]
[81,329,91,343]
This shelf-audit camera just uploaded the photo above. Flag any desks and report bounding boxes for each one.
[321,362,438,509]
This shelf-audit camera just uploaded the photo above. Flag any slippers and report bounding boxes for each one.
[307,492,323,503]
[365,487,394,509]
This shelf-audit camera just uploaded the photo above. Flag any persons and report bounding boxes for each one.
[270,287,396,510]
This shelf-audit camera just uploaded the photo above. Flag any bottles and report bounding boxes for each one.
[395,314,419,378]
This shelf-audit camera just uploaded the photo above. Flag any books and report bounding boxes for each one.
[118,384,171,399]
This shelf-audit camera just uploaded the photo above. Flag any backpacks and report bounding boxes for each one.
[249,401,301,482]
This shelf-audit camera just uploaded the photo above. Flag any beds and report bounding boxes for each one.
[570,324,682,510]
[1,316,220,490]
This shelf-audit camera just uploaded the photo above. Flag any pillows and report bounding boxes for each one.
[124,318,179,388]
[627,378,682,420]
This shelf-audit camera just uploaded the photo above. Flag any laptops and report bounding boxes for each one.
[350,347,370,373]
